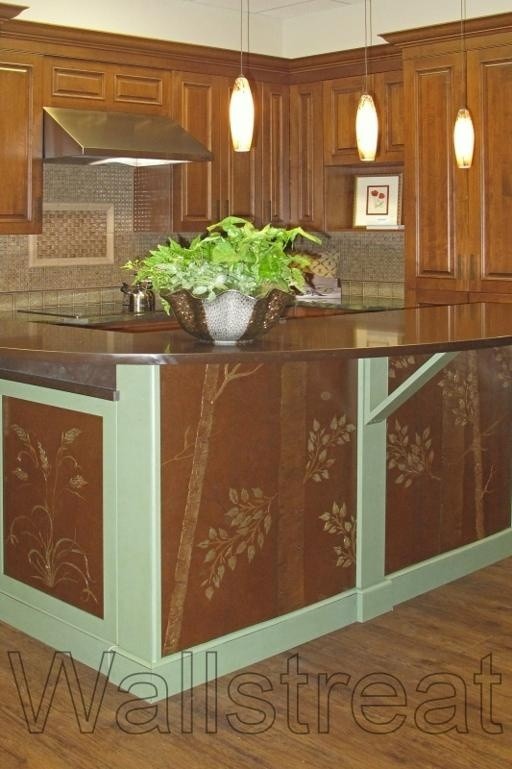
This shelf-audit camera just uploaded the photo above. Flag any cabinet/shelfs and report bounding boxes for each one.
[264,81,324,232]
[403,45,512,309]
[324,70,404,167]
[43,54,172,116]
[135,71,263,234]
[0,48,43,234]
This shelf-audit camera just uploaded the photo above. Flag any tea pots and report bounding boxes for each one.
[118,276,158,313]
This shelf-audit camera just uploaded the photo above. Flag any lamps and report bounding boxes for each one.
[228,0,254,153]
[453,0,474,169]
[356,0,379,161]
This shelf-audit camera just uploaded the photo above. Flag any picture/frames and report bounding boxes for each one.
[352,173,403,229]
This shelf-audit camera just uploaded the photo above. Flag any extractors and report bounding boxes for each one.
[42,106,214,167]
